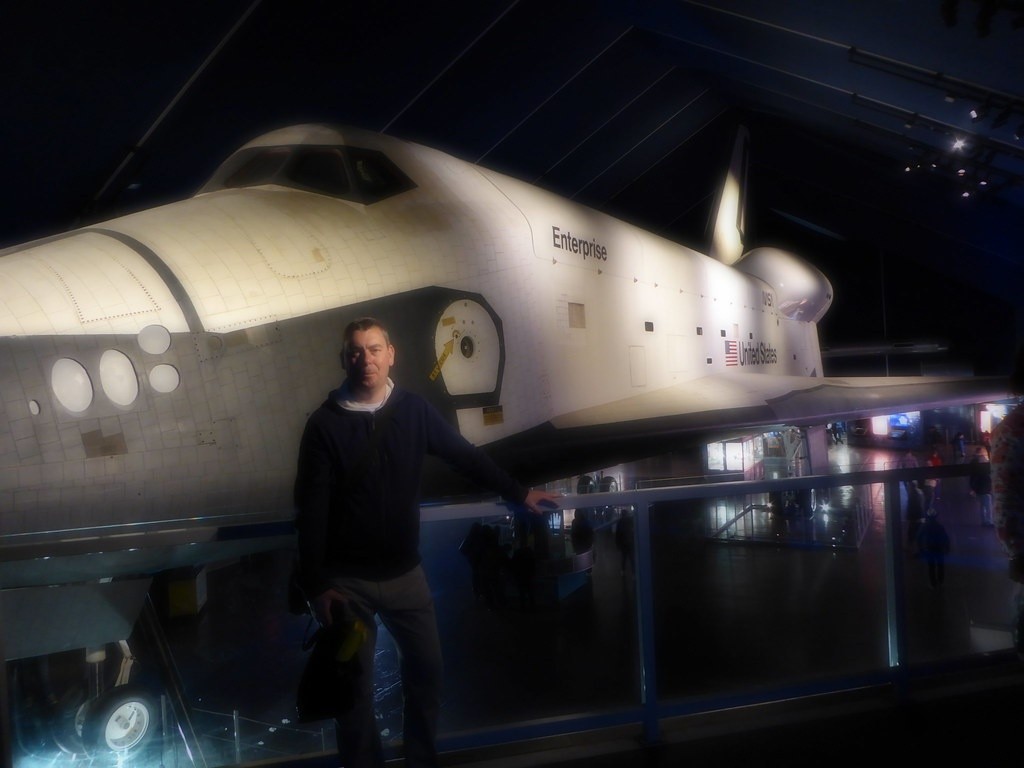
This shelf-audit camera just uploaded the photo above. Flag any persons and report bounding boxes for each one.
[970,474,994,529]
[898,449,920,494]
[918,509,949,583]
[921,479,935,507]
[906,480,923,545]
[956,432,966,463]
[926,450,943,501]
[983,432,991,459]
[616,510,634,574]
[927,426,943,454]
[295,319,562,768]
[832,423,843,444]
[467,522,486,597]
[573,510,594,575]
[510,516,548,551]
[971,448,987,463]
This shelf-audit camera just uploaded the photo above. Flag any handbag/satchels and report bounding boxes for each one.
[297,598,364,724]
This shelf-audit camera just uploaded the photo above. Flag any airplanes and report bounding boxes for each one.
[0,119,1024,672]
